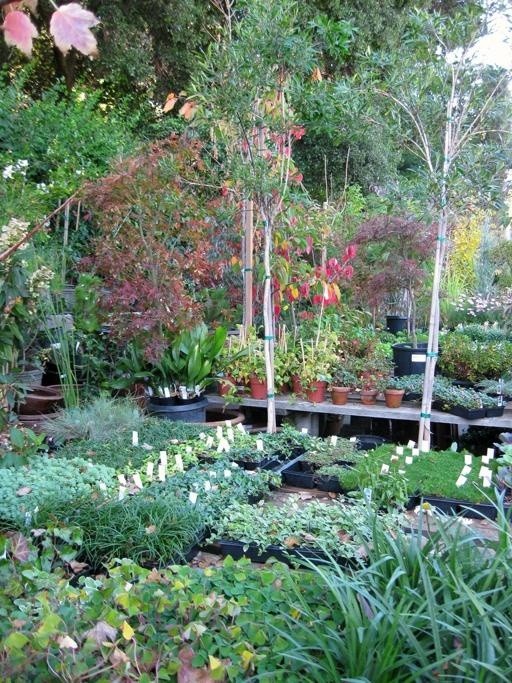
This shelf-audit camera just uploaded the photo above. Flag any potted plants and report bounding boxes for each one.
[64,315,511,582]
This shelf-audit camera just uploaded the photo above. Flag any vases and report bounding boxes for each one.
[12,362,83,426]
